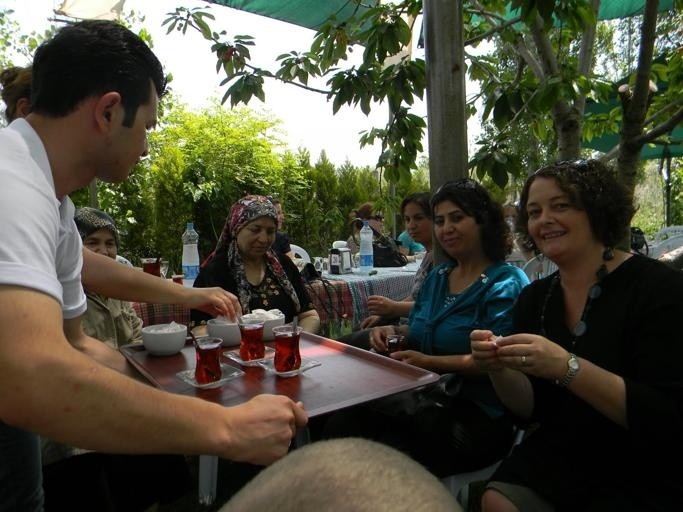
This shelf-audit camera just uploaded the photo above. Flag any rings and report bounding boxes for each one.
[521,356,526,362]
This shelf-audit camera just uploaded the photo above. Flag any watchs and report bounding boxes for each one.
[559,353,580,387]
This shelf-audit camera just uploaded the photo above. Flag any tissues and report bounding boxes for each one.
[328,240,352,275]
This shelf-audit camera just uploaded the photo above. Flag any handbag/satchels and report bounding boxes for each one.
[372,238,408,267]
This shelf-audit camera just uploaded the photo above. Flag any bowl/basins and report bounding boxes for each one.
[207,317,262,344]
[243,312,285,343]
[139,322,188,356]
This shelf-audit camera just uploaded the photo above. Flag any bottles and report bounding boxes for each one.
[312,256,330,276]
[357,220,374,274]
[179,222,201,289]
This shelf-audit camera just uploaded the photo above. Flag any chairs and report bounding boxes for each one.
[440,429,524,511]
[329,237,349,251]
[196,423,311,508]
[521,226,682,284]
[284,244,311,267]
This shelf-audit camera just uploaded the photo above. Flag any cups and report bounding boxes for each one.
[270,325,304,370]
[140,257,161,277]
[171,272,184,287]
[235,318,267,356]
[384,333,405,356]
[190,337,223,382]
[413,253,424,269]
[160,259,170,281]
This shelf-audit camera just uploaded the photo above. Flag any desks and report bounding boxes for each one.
[127,266,419,339]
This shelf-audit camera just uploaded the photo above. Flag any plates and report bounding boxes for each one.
[174,362,244,392]
[258,353,322,378]
[222,343,274,367]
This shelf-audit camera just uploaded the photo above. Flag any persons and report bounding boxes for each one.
[36,207,196,506]
[270,197,296,268]
[0,66,25,89]
[349,188,434,332]
[185,194,323,334]
[222,434,465,512]
[0,18,310,506]
[501,201,520,233]
[2,64,43,121]
[327,178,534,485]
[395,228,427,264]
[467,161,682,512]
[344,203,385,259]
